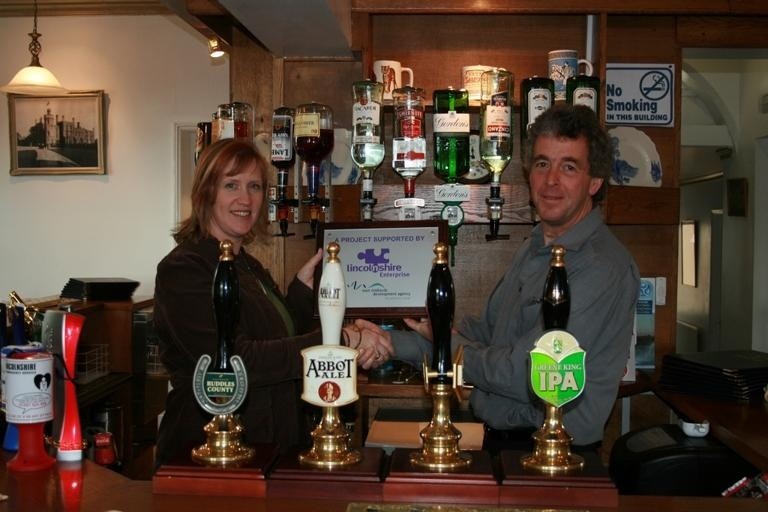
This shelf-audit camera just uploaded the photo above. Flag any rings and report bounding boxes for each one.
[376,353,381,360]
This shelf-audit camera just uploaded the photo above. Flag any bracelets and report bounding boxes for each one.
[353,323,362,349]
[342,329,350,348]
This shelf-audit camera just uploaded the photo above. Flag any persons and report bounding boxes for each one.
[349,103,642,463]
[152,135,396,465]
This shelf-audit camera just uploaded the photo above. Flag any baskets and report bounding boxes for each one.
[75,343,110,383]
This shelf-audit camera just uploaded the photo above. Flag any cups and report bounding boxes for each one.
[461,65,510,99]
[372,60,414,101]
[547,49,593,100]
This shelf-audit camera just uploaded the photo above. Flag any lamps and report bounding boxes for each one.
[1,0,69,96]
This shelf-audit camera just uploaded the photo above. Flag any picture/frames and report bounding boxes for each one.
[679,220,698,287]
[7,90,106,176]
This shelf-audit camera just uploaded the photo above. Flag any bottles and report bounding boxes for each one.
[193,103,254,172]
[293,104,336,198]
[350,70,606,198]
[272,107,295,200]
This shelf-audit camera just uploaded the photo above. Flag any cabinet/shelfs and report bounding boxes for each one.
[41,297,158,473]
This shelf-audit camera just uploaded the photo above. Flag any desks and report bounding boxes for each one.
[648,373,767,476]
[356,378,477,447]
